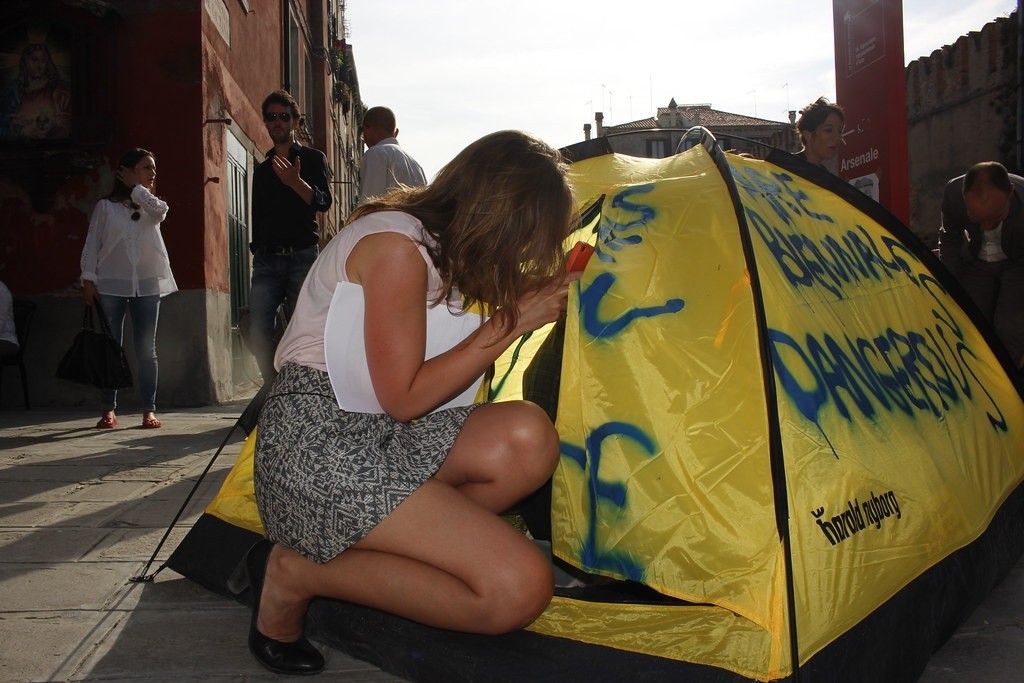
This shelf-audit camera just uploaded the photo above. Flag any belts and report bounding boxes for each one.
[257,244,314,255]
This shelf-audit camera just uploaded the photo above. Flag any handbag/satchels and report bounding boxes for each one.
[56,296,133,390]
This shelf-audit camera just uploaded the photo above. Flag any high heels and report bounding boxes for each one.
[226,539,325,675]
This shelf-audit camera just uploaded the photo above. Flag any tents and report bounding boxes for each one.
[141,128,1024,683]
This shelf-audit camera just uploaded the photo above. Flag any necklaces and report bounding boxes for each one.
[124,198,141,222]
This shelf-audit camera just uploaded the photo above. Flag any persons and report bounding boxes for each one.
[228,131,583,675]
[248,89,334,386]
[81,148,178,430]
[0,283,19,366]
[792,98,848,167]
[938,161,1024,381]
[356,106,428,200]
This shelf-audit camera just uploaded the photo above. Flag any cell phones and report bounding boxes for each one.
[564,240,595,273]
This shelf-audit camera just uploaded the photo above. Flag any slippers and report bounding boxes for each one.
[96,410,117,429]
[142,417,161,428]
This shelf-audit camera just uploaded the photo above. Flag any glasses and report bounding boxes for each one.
[130,202,141,222]
[266,111,290,122]
